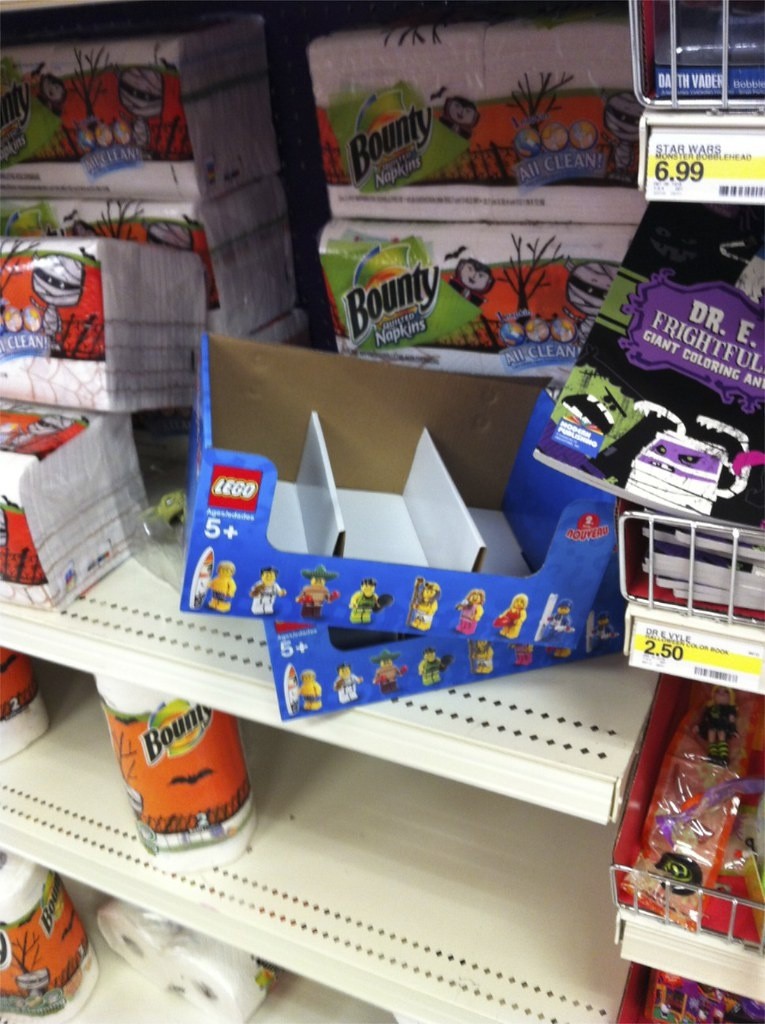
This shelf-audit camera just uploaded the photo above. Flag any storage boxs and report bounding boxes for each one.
[261,549,628,723]
[180,332,620,642]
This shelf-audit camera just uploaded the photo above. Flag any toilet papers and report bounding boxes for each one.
[93,673,258,876]
[0,845,102,1024]
[96,896,289,1024]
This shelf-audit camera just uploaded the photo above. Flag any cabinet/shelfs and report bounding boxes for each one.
[615,109,765,1004]
[0,528,660,1024]
